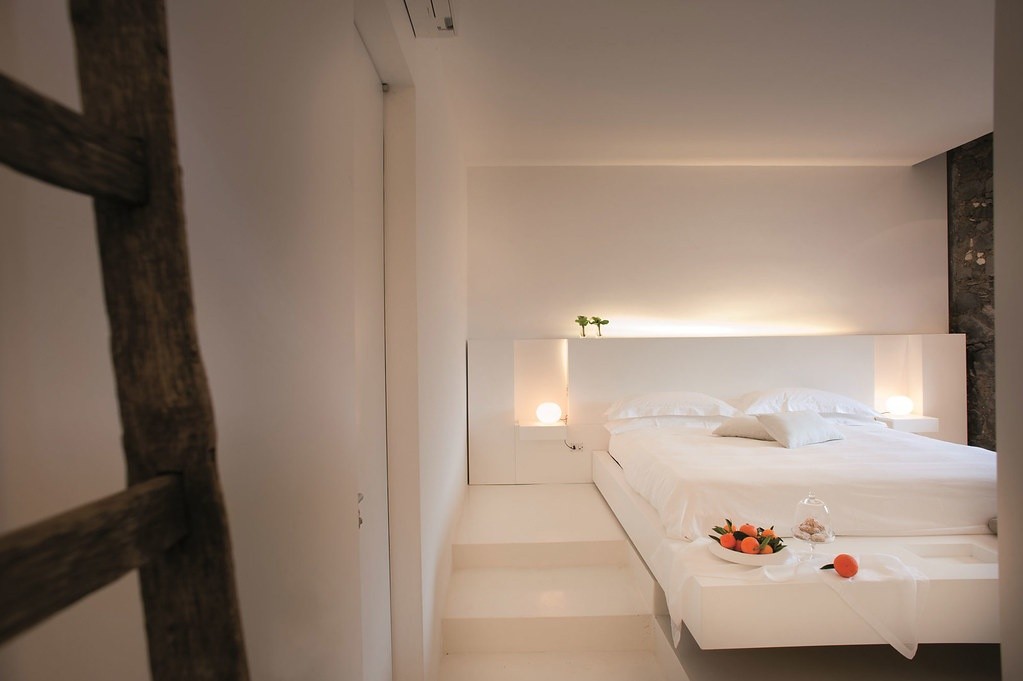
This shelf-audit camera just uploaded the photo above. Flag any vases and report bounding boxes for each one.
[579,327,588,338]
[595,328,605,338]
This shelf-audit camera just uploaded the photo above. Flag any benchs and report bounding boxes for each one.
[675,534,1000,652]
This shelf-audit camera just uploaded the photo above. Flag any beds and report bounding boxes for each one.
[609,417,997,542]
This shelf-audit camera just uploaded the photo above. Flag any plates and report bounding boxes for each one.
[709,530,791,566]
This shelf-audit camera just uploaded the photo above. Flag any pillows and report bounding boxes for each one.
[739,386,888,419]
[755,410,846,449]
[711,415,777,441]
[602,390,739,419]
[603,416,721,436]
[821,413,889,429]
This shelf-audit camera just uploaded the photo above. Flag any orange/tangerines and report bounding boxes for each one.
[833,553,858,577]
[720,523,776,554]
